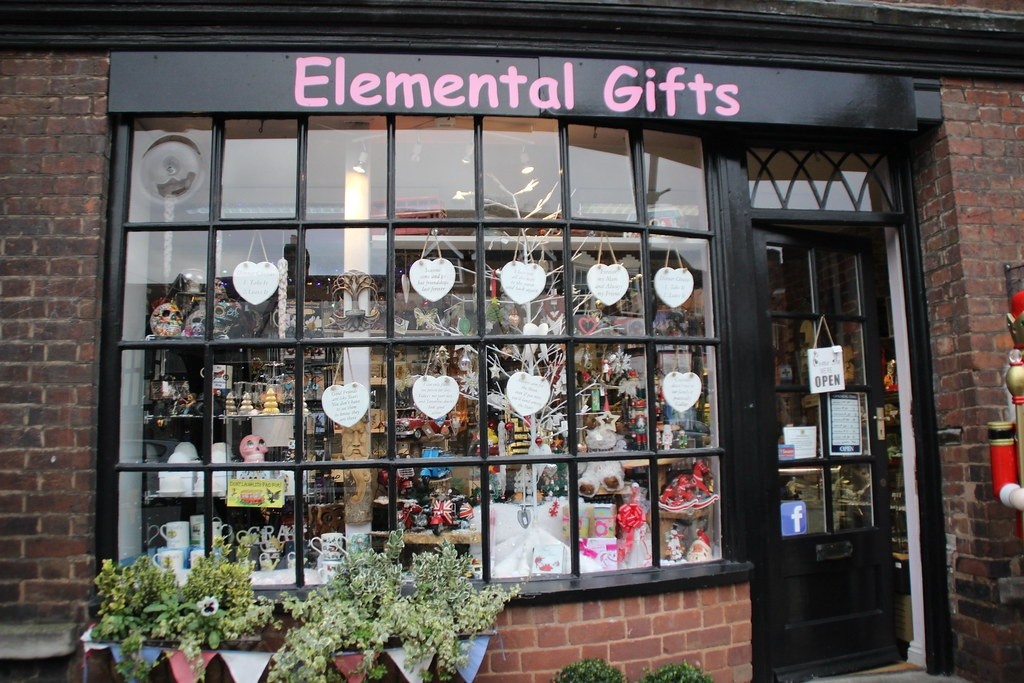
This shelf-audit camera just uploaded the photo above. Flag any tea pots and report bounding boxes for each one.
[259,553,280,571]
[287,552,308,571]
[258,535,285,558]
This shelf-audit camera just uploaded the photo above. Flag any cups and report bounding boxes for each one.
[152,516,233,575]
[200,365,233,390]
[310,532,371,584]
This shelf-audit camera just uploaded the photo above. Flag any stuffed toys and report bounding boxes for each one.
[575,425,630,499]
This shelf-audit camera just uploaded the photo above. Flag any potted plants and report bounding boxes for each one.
[91,533,520,683]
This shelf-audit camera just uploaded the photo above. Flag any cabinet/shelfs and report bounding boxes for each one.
[322,327,449,451]
[138,308,336,568]
